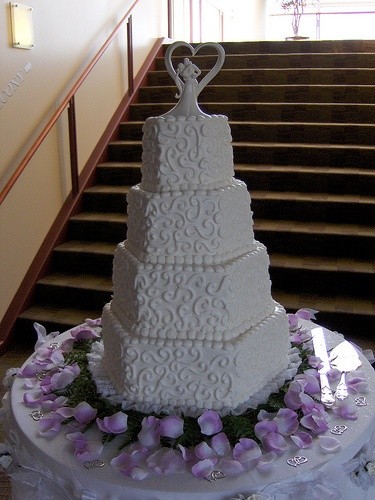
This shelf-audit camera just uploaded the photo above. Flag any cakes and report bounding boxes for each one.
[0,40,374,498]
